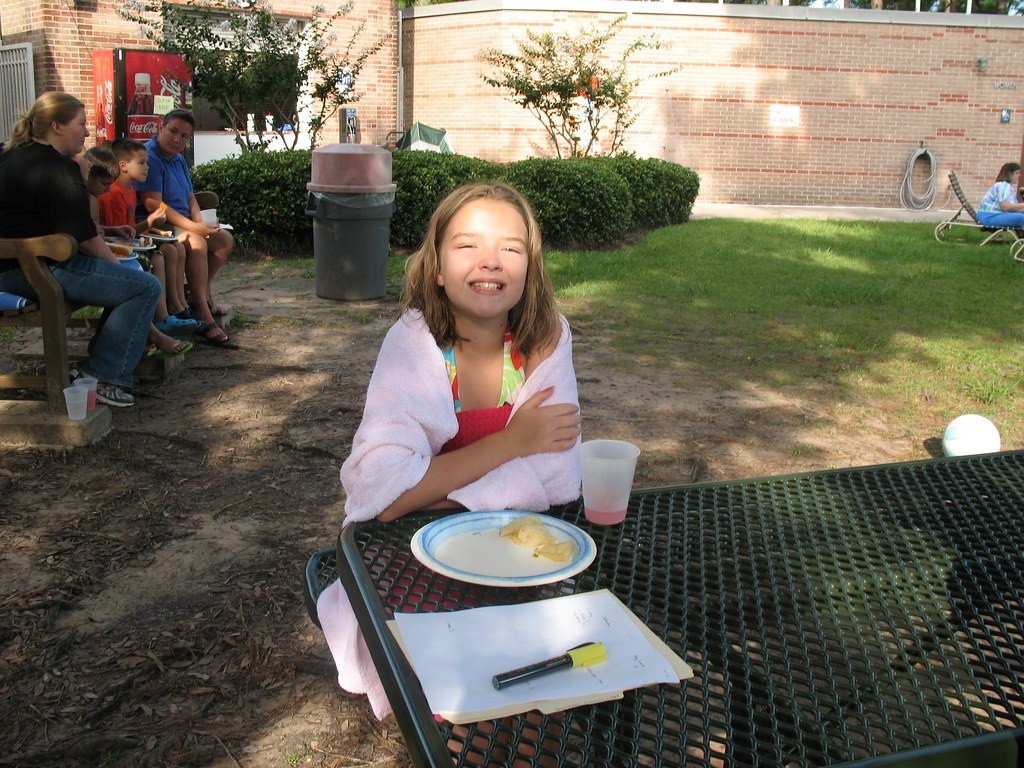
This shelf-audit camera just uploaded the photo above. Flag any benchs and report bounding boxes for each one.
[0,189,220,415]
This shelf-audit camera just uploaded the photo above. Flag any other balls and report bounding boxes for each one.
[942,413,1002,460]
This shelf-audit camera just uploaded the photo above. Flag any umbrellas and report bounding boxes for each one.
[394,123,453,153]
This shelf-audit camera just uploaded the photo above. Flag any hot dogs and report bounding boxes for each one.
[146,227,168,237]
[140,237,153,247]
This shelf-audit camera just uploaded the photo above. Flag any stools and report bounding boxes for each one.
[300,548,340,633]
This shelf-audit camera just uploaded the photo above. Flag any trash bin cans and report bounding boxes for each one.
[304,144,396,301]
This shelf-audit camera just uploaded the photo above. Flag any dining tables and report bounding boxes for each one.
[336,446,1024,768]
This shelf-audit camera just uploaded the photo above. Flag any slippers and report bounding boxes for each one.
[166,304,198,320]
[154,315,202,337]
[139,338,160,357]
[159,341,194,357]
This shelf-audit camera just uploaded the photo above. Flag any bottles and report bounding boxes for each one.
[95,85,106,146]
[126,72,160,145]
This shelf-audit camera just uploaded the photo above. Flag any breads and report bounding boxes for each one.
[106,243,134,255]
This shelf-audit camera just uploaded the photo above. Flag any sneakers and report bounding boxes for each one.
[67,370,134,407]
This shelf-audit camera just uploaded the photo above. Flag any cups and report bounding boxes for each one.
[200,208,218,227]
[74,377,98,411]
[63,387,88,420]
[246,113,255,132]
[579,439,642,525]
[265,116,273,132]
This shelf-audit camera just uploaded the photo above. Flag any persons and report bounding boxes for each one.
[66,145,193,358]
[0,91,162,407]
[975,163,1024,227]
[130,110,233,345]
[80,145,199,335]
[95,137,206,333]
[315,183,584,768]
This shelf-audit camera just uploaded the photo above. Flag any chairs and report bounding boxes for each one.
[934,171,1024,248]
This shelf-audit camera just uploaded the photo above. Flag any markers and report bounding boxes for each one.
[492,641,607,693]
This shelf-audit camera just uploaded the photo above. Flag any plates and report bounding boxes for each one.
[139,233,178,243]
[131,239,156,251]
[112,251,139,261]
[410,510,597,587]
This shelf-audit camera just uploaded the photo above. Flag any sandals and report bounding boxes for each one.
[204,300,229,316]
[196,320,230,346]
